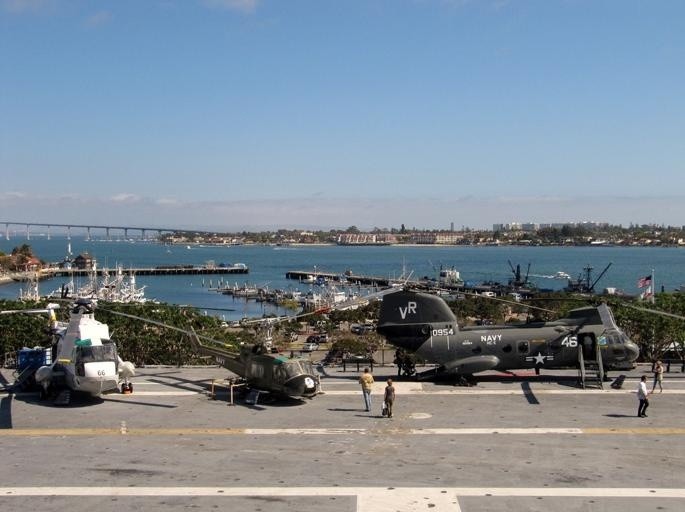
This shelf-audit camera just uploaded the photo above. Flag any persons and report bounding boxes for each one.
[383,378,396,418]
[636,375,650,418]
[394,346,407,376]
[651,359,664,394]
[359,367,375,412]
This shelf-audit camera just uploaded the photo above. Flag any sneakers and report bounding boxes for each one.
[364,408,372,412]
[649,389,664,394]
[387,412,393,418]
[638,413,649,419]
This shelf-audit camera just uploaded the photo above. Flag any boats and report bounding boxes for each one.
[554,272,569,279]
[217,281,359,309]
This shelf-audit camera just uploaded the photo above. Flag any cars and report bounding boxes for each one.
[284,331,328,352]
[350,323,374,334]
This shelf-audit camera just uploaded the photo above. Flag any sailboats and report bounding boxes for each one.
[16,259,147,303]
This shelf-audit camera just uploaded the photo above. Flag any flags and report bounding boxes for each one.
[636,273,652,290]
[638,286,655,303]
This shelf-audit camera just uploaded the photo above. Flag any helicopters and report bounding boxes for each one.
[0,299,139,399]
[188,312,326,405]
[376,290,673,390]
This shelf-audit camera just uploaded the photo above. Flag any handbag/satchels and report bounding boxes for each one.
[382,408,388,415]
[381,401,386,410]
